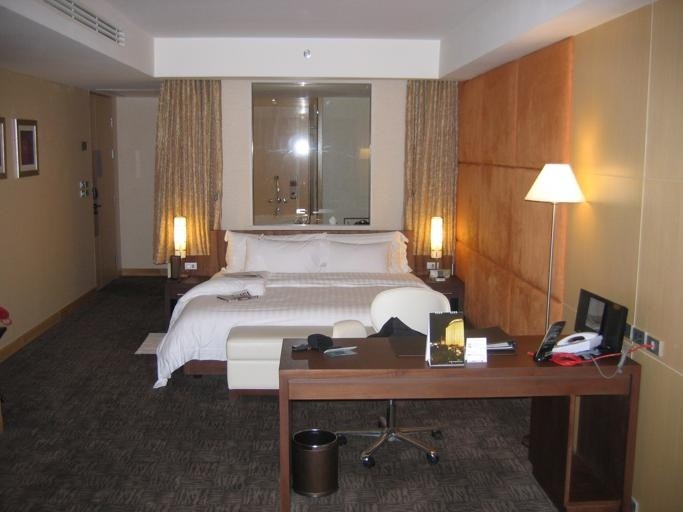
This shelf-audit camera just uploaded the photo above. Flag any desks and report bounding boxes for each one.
[277,335,641,512]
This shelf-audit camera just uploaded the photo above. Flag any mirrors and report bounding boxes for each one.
[251,81,371,226]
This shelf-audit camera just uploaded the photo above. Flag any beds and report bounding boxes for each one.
[170,229,451,379]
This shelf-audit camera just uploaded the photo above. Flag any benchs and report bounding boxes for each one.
[225,325,376,400]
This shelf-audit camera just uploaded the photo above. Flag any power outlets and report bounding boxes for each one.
[645,335,660,355]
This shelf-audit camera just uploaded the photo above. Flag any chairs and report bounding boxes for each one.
[337,288,452,469]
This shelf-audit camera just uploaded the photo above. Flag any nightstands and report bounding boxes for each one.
[418,275,465,315]
[165,275,211,315]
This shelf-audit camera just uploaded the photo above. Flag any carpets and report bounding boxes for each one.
[134,332,168,354]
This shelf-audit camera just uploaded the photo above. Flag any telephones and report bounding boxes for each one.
[551,331,604,354]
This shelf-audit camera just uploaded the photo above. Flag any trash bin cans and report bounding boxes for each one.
[291,428,347,499]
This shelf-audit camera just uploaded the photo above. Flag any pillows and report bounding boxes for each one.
[221,231,412,277]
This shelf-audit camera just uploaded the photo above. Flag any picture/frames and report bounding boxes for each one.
[0,117,39,179]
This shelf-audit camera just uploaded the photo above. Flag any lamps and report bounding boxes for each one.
[523,163,586,335]
[430,217,445,260]
[173,216,187,258]
